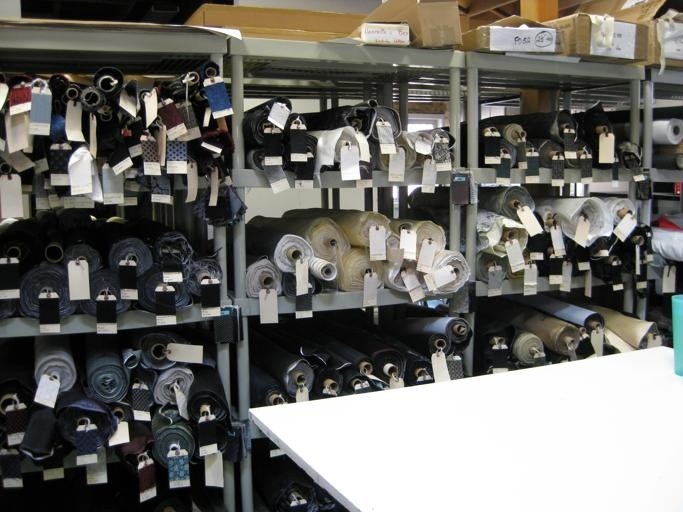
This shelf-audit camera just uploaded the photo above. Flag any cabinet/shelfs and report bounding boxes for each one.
[0,25,683,512]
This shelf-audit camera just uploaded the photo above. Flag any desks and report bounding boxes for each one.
[246,346,683,512]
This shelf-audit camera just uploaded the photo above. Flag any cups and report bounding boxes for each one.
[671,294,683,377]
